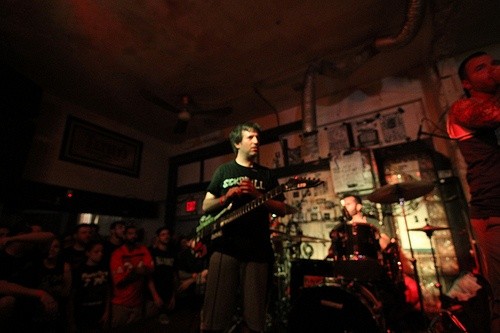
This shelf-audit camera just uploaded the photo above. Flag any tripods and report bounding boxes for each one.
[424,231,468,333]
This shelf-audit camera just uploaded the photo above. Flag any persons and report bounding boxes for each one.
[328,195,369,256]
[445,52,500,333]
[0,219,210,333]
[202,122,286,333]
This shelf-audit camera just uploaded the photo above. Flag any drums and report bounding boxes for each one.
[277,236,329,242]
[283,278,386,333]
[331,223,381,260]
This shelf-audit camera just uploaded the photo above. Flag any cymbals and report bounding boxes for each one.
[285,202,296,215]
[408,225,450,234]
[367,182,435,205]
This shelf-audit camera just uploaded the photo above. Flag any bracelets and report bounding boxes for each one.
[219,196,225,206]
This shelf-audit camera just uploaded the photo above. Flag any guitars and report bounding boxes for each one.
[190,176,325,258]
